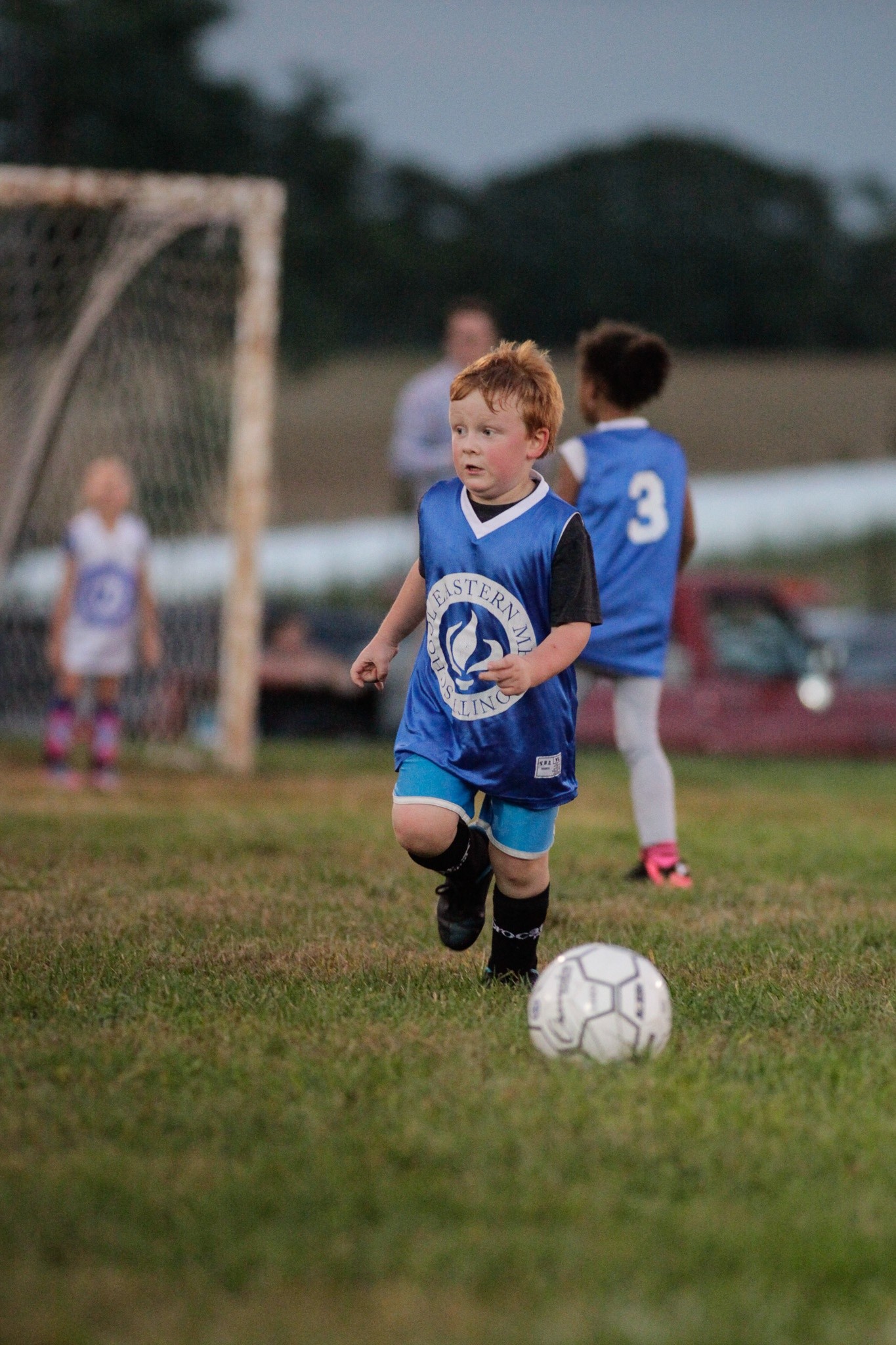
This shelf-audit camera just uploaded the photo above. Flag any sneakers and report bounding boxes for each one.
[481,958,538,990]
[435,819,496,952]
[622,856,694,891]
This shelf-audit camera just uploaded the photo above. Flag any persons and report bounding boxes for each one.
[350,336,605,989]
[554,316,699,892]
[389,298,557,506]
[41,454,161,794]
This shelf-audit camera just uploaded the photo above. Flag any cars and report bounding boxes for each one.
[235,606,430,743]
[576,574,896,759]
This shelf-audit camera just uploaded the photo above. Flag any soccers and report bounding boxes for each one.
[526,944,673,1066]
